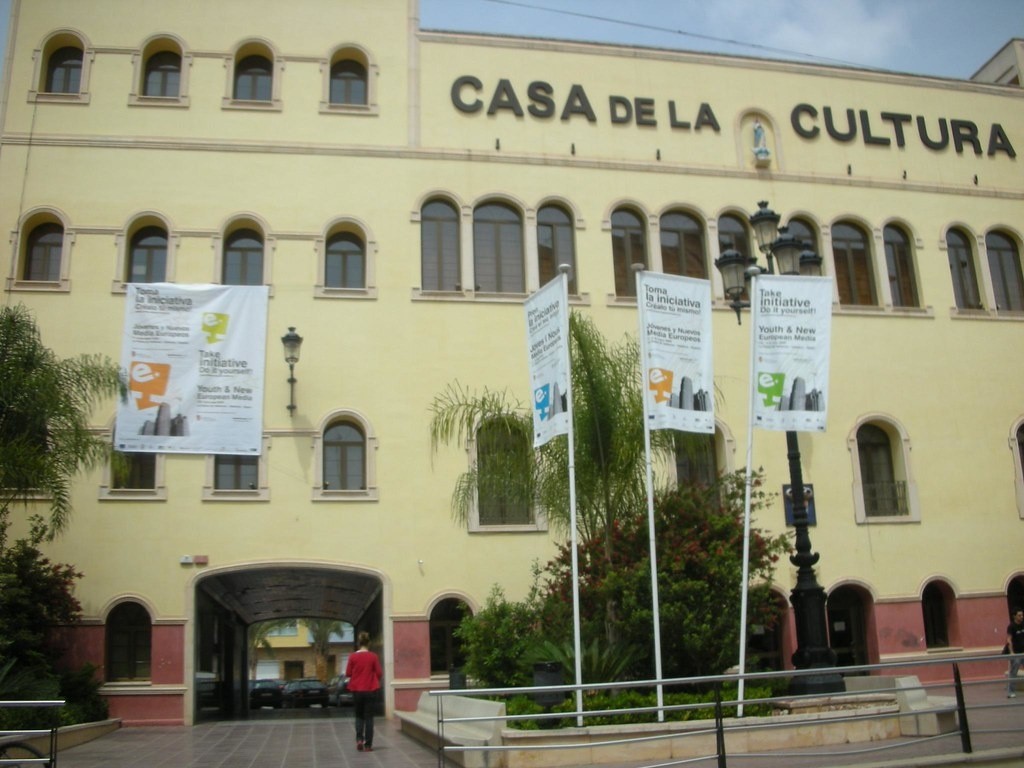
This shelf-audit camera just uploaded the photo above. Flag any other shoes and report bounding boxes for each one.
[364,747,371,751]
[357,740,364,751]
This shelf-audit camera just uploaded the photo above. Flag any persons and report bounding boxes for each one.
[345,632,383,752]
[1002,610,1024,698]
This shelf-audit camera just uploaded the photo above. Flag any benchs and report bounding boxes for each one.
[843,674,958,736]
[394,690,507,768]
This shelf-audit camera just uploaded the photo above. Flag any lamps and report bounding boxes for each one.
[280,326,304,417]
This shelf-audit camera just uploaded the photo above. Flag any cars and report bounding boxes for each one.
[249,674,355,708]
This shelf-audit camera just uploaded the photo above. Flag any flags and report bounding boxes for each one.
[634,269,716,435]
[749,274,831,434]
[521,273,573,448]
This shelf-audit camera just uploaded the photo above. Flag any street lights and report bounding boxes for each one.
[714,200,847,694]
